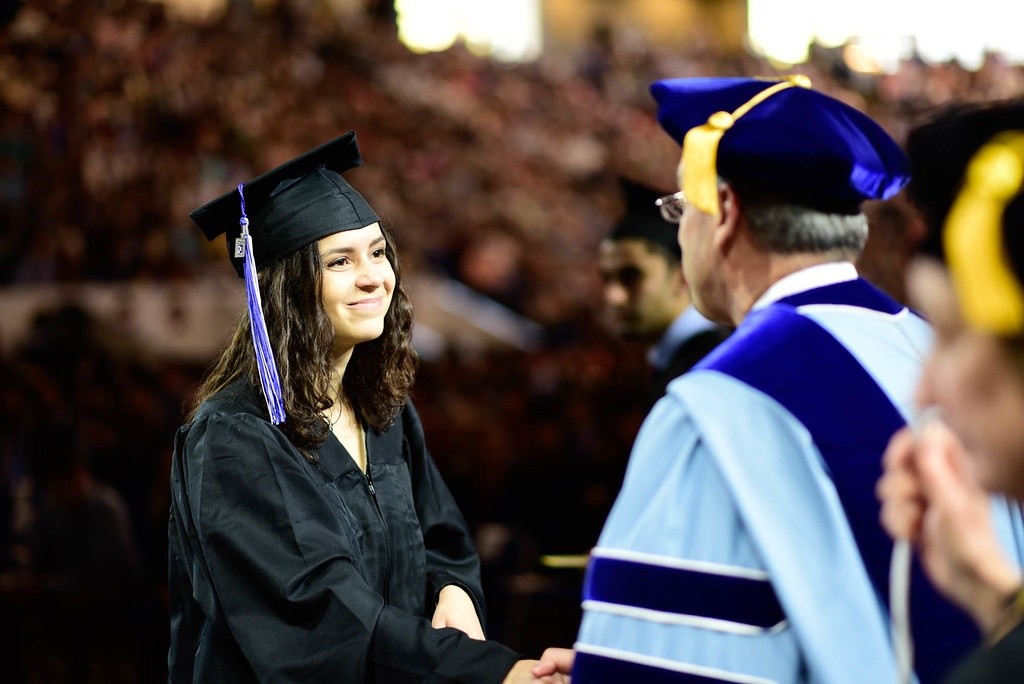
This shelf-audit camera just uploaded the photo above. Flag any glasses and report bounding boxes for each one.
[656,191,687,222]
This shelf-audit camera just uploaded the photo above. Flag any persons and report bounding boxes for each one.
[163,128,574,684]
[0,0,1024,684]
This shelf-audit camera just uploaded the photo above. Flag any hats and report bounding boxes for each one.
[189,129,381,425]
[606,173,684,261]
[647,73,913,214]
[901,95,1024,340]
